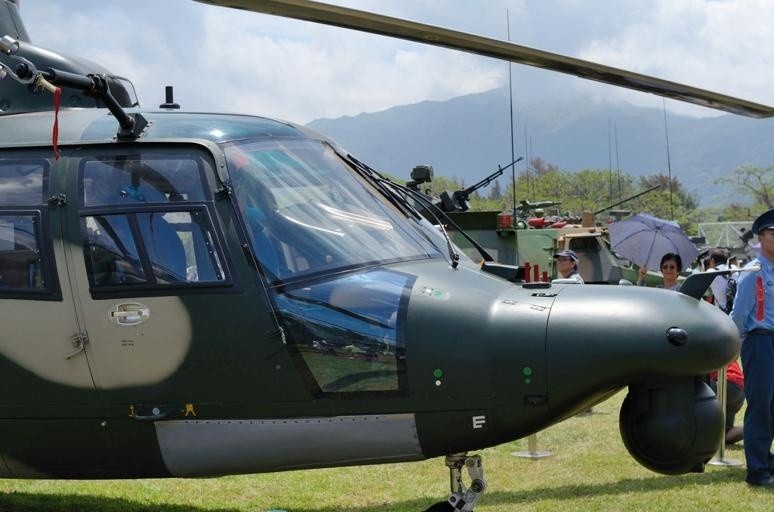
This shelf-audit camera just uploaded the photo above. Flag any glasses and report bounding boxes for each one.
[661,264,676,269]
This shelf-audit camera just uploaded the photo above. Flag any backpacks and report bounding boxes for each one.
[715,268,737,315]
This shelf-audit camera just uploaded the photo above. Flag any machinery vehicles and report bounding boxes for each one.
[404,154,664,314]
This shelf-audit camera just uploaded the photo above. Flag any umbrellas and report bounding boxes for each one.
[607,212,698,272]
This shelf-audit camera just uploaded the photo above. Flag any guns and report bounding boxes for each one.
[454,157,523,202]
[512,199,562,212]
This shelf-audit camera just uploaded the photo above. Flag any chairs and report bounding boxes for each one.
[101,185,286,282]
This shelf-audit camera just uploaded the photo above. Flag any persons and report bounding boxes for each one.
[710,361,747,444]
[635,251,681,290]
[556,251,584,285]
[702,251,735,314]
[728,206,774,491]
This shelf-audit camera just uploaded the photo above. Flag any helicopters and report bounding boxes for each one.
[0,1,774,512]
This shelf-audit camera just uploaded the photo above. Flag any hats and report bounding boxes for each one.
[554,249,579,262]
[752,209,774,235]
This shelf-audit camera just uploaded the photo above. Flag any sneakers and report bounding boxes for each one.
[749,476,774,487]
